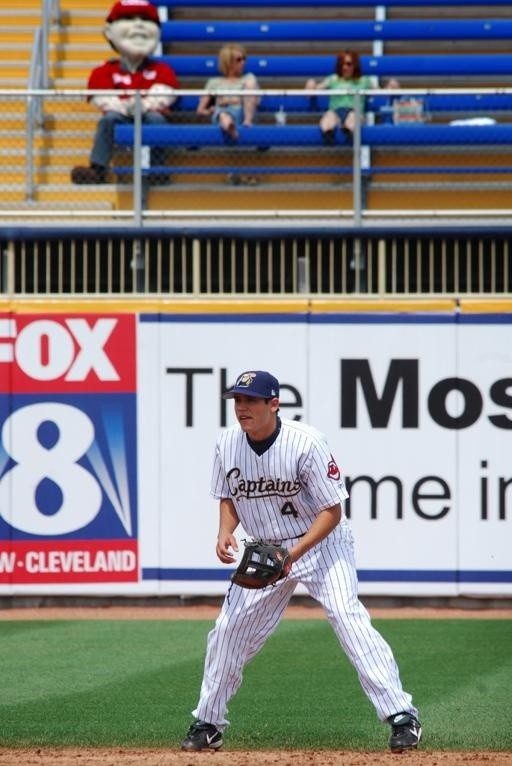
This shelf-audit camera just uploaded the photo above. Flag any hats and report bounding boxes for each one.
[222,370,279,401]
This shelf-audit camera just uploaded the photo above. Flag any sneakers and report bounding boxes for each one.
[181,718,223,751]
[389,711,422,752]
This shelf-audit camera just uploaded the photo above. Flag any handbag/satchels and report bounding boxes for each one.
[393,98,423,124]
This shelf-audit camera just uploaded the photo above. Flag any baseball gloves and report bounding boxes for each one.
[231,539,292,591]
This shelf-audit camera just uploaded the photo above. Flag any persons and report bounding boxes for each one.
[181,372,422,754]
[303,49,400,138]
[73,0,180,184]
[196,41,262,137]
